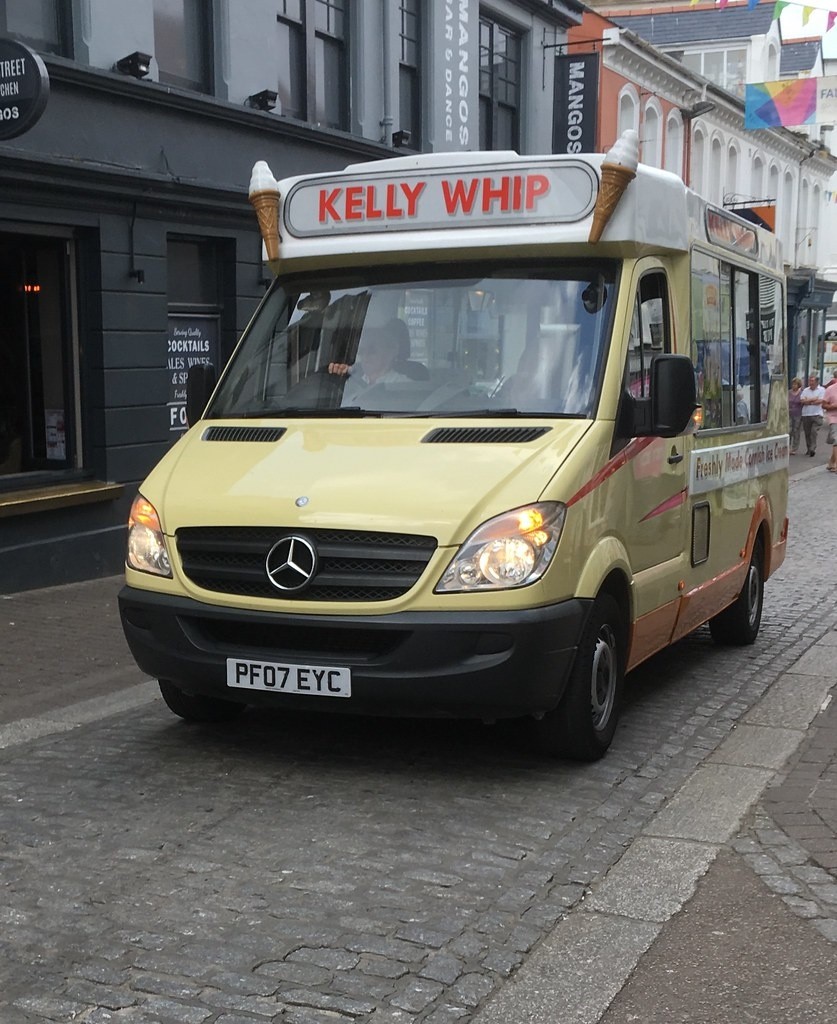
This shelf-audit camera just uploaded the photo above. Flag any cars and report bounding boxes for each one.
[699,338,772,421]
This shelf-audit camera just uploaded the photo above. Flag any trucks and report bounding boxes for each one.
[111,128,791,768]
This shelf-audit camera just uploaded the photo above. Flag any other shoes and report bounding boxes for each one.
[789,449,796,454]
[827,459,837,472]
[805,449,815,457]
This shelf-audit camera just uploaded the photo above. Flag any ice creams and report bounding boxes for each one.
[587,129,641,241]
[249,158,284,261]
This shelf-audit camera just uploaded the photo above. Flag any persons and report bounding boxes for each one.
[789,370,837,472]
[735,392,751,426]
[327,330,415,409]
